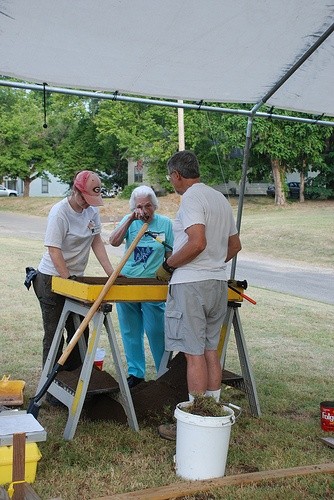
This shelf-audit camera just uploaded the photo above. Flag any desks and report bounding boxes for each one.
[29,276,262,440]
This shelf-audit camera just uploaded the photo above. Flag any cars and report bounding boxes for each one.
[267,182,320,200]
[0,185,19,197]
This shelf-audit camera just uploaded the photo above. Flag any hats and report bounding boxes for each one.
[74,171,104,206]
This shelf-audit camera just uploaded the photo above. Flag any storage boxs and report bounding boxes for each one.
[0,412,47,484]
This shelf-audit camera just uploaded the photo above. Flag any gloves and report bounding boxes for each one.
[155,258,175,281]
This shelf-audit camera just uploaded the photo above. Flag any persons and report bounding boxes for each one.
[109,186,175,388]
[31,171,127,407]
[157,151,242,441]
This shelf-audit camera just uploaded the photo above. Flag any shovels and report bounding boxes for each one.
[27,222,149,419]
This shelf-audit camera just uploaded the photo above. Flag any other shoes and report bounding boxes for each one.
[127,375,145,387]
[159,424,176,440]
[46,393,61,405]
